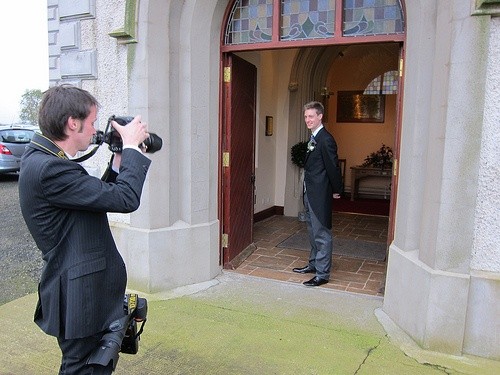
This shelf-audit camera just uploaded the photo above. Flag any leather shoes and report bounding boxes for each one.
[303,276,328,286]
[293,265,316,274]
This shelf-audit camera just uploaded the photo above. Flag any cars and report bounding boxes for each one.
[0,126,35,174]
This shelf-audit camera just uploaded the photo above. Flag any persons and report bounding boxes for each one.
[18,86,152,375]
[293,102,342,286]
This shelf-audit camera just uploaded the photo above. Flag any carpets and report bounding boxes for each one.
[275,232,387,262]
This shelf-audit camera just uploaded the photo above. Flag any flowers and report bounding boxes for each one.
[307,136,317,151]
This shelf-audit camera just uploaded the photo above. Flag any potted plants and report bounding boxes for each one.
[289,142,309,222]
[362,144,393,168]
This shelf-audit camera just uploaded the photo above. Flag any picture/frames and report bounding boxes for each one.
[336,90,385,123]
[265,116,273,136]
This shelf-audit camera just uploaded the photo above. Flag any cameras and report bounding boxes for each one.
[91,116,164,155]
[85,293,147,375]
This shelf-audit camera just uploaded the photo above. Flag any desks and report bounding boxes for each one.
[350,165,393,203]
